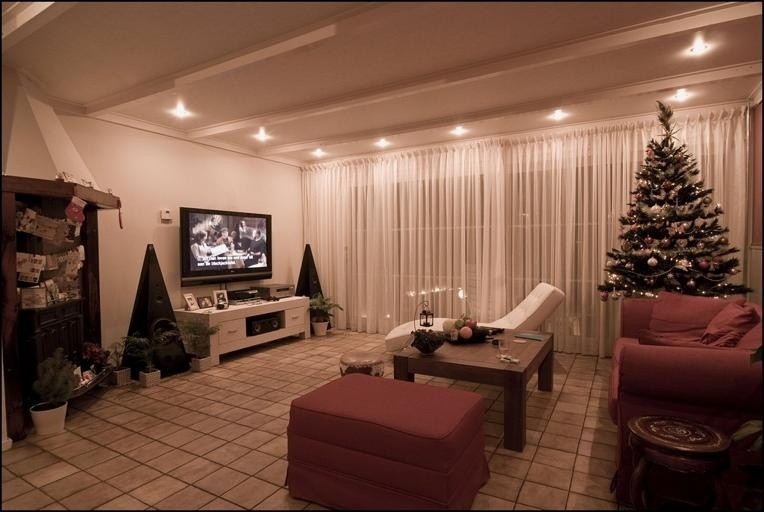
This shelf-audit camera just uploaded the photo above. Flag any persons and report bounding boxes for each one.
[200,297,212,307]
[188,298,194,306]
[217,293,226,303]
[191,217,265,268]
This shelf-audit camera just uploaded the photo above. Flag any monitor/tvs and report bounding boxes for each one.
[180,207,272,288]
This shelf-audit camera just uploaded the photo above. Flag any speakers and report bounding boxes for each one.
[120,244,191,381]
[295,244,331,335]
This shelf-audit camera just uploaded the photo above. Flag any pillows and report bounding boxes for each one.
[645,288,747,348]
[699,301,761,349]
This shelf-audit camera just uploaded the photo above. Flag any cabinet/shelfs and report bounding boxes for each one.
[173,294,310,369]
[0,173,121,439]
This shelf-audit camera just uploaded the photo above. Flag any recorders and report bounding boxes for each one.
[248,315,280,336]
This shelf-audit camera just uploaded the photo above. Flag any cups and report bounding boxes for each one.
[498,340,509,356]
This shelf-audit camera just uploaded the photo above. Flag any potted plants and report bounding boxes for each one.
[105,339,130,387]
[120,331,178,387]
[24,345,76,437]
[305,293,343,338]
[175,317,219,372]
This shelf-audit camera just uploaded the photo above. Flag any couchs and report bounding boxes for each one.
[281,370,493,512]
[608,289,764,504]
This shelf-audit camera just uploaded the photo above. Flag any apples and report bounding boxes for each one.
[443,317,477,339]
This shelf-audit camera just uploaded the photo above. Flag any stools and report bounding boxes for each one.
[627,412,731,511]
[337,350,385,377]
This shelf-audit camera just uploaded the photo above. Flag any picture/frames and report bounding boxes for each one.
[195,296,213,309]
[182,293,199,311]
[212,289,229,307]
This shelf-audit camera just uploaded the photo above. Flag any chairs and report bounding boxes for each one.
[384,280,567,353]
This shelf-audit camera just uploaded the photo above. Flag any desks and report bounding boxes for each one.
[391,327,555,452]
